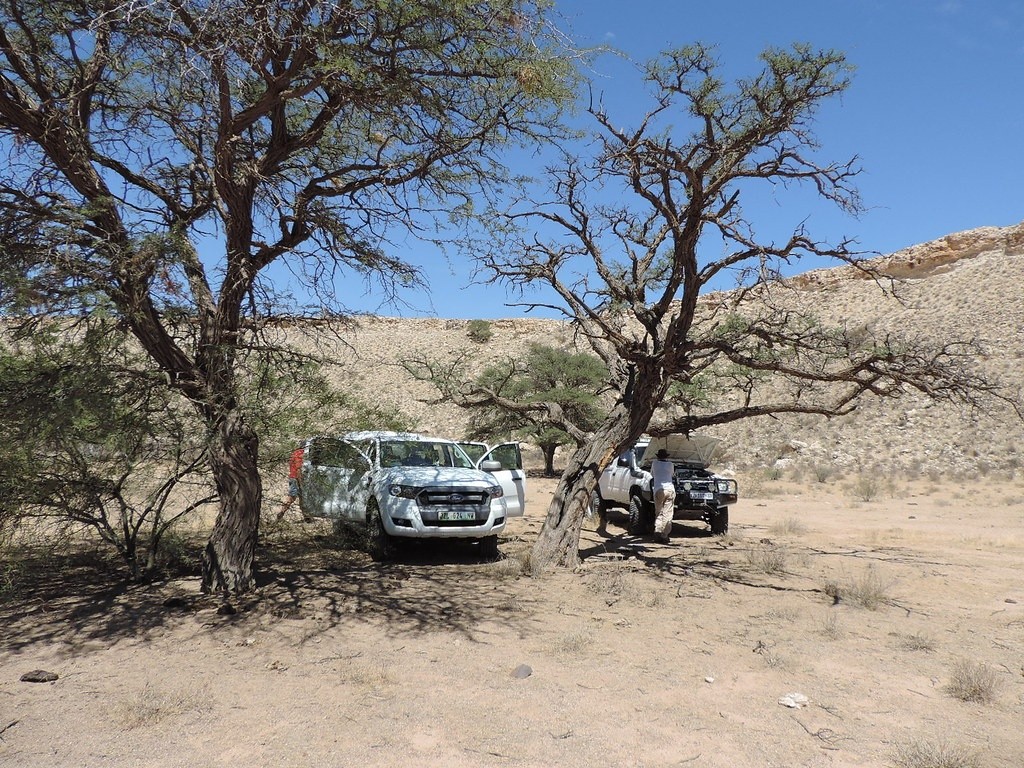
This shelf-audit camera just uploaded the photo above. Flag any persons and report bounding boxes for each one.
[276,440,314,524]
[650,449,676,544]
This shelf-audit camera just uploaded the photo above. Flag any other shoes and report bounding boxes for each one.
[660,538,670,544]
[655,532,662,542]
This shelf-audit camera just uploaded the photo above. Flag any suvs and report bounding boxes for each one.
[301,431,526,552]
[568,433,738,535]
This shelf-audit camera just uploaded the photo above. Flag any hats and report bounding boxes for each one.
[655,449,670,459]
